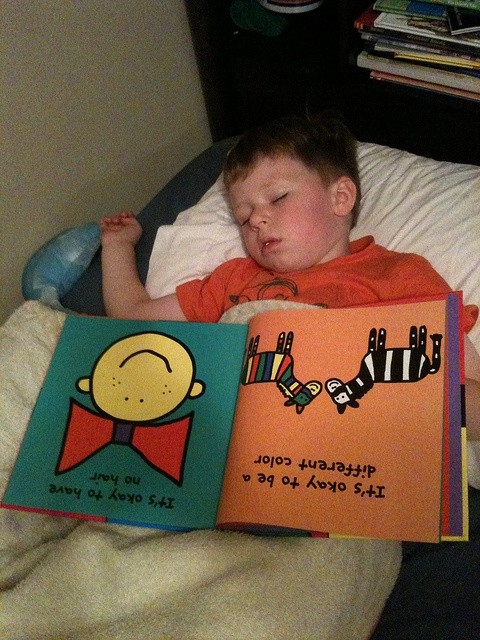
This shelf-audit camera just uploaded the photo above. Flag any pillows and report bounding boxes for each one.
[144,139,480,359]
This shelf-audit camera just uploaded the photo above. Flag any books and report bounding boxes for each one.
[0,288,470,544]
[353,1,480,100]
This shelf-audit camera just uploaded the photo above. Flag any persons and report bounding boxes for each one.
[98,111,480,442]
